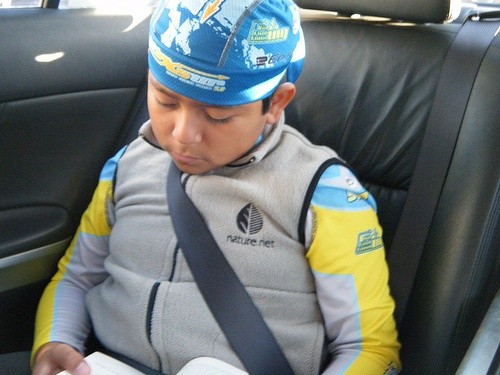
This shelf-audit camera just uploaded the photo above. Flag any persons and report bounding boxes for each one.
[29,0,402,375]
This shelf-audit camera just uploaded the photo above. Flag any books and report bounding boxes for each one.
[56,351,250,375]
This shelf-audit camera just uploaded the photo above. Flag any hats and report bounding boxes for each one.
[148,0,306,106]
